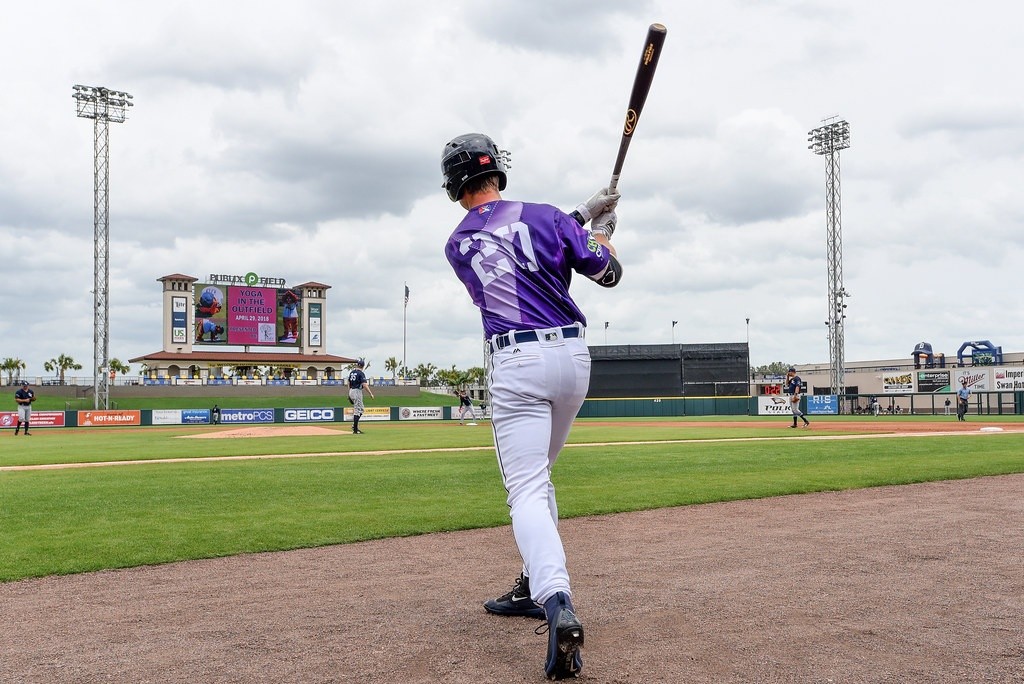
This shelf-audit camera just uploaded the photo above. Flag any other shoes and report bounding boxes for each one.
[352,425,361,434]
[24,433,32,436]
[15,430,19,435]
[957,414,960,421]
[789,425,797,428]
[803,422,809,427]
[460,422,463,424]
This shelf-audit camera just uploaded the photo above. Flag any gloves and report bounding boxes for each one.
[576,187,621,226]
[590,211,617,240]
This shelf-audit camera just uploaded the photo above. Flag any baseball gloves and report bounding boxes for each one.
[792,397,800,403]
[454,390,460,397]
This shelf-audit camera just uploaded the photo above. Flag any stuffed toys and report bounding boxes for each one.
[197,285,223,314]
[279,290,300,338]
[196,319,224,341]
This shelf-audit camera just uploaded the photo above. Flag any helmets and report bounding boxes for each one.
[440,132,511,202]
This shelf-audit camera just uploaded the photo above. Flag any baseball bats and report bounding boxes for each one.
[603,23,667,214]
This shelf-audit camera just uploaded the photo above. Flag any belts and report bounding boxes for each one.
[490,327,579,355]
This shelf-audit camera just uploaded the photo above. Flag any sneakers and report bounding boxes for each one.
[542,591,584,681]
[483,572,546,620]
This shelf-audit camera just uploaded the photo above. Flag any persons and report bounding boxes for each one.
[785,368,810,428]
[440,132,621,679]
[15,381,36,436]
[455,389,476,424]
[856,404,900,415]
[871,395,879,417]
[957,381,972,421]
[478,401,486,421]
[347,360,374,435]
[944,398,952,416]
[213,404,221,424]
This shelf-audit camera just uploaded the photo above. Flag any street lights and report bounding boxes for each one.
[808,114,851,415]
[71,83,134,410]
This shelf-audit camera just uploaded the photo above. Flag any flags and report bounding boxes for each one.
[604,322,609,329]
[405,286,409,307]
[746,319,750,324]
[673,320,678,328]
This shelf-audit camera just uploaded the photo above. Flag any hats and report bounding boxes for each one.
[461,389,465,392]
[788,368,795,372]
[358,361,364,366]
[22,381,29,387]
